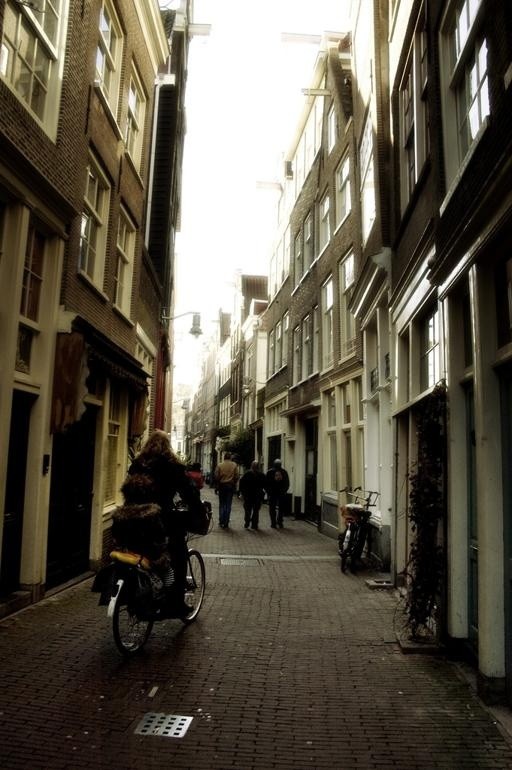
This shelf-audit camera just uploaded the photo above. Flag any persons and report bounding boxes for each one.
[185,463,204,502]
[239,459,266,531]
[213,453,240,531]
[90,428,210,619]
[264,458,290,531]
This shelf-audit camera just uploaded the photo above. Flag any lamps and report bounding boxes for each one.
[164,308,203,339]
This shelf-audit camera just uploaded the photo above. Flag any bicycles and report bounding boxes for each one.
[336,486,381,573]
[107,499,213,654]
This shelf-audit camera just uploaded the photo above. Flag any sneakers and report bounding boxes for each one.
[219,522,283,529]
